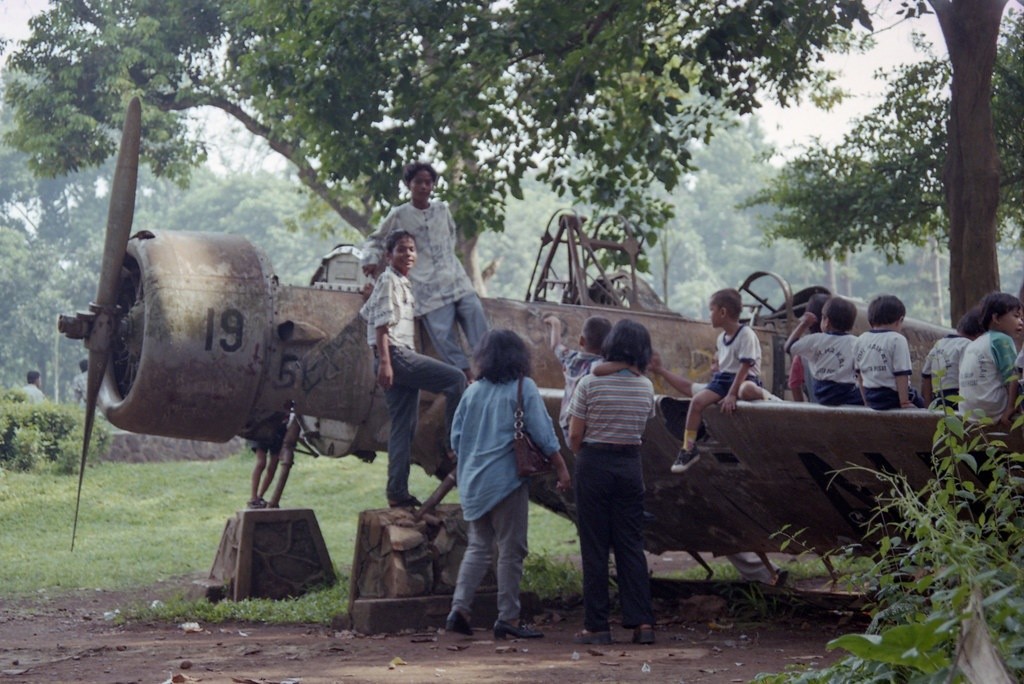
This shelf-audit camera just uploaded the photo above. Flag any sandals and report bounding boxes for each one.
[388,495,422,507]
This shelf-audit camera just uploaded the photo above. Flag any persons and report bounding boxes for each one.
[784,297,864,405]
[74,359,89,403]
[921,308,983,411]
[360,162,490,382]
[852,296,924,409]
[650,348,782,402]
[671,288,761,475]
[359,231,467,509]
[544,313,641,447]
[445,330,569,639]
[959,293,1024,425]
[568,318,656,644]
[22,371,44,401]
[789,292,829,401]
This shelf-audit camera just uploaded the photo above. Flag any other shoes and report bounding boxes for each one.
[258,497,268,507]
[247,500,261,508]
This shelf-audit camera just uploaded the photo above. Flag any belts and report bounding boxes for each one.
[583,442,640,453]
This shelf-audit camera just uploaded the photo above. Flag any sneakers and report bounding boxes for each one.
[670,444,700,474]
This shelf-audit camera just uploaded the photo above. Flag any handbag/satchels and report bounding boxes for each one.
[514,372,567,477]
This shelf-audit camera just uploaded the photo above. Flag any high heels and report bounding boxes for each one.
[494,620,544,640]
[572,626,611,645]
[633,624,655,643]
[445,610,473,635]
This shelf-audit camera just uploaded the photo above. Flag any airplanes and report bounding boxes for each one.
[58,96,1024,616]
